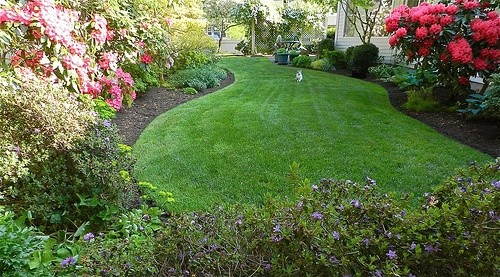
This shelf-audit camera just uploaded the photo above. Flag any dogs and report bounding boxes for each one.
[296,69,303,82]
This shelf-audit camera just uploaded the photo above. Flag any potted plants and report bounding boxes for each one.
[273,48,286,62]
[287,50,299,61]
[277,48,289,65]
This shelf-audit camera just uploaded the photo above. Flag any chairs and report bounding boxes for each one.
[276,41,301,50]
